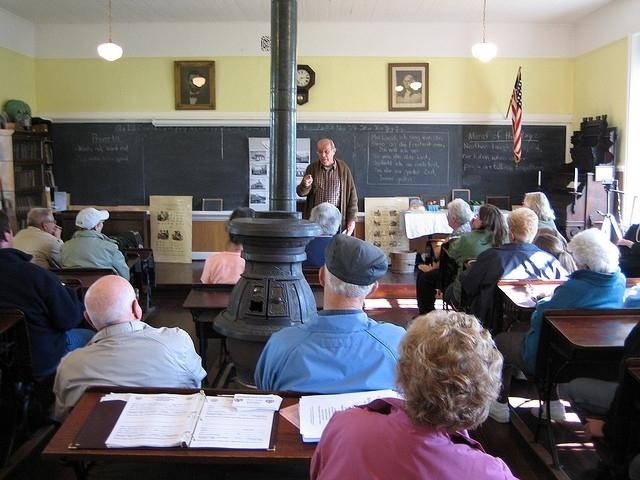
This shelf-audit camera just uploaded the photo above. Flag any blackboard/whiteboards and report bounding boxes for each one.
[31,116,571,221]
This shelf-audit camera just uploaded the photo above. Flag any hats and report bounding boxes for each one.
[75,208,110,229]
[325,234,388,285]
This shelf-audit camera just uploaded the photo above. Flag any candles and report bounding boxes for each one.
[537,171,542,186]
[574,168,579,191]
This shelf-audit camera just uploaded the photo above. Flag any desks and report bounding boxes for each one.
[402,208,513,265]
[183,283,322,385]
[40,386,319,480]
[1,248,155,466]
[496,278,639,471]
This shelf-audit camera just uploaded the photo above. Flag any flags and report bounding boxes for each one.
[510,69,522,165]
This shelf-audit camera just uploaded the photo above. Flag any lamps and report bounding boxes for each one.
[96,0,124,60]
[471,0,497,61]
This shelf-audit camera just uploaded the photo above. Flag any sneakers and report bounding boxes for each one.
[531,402,565,419]
[489,401,510,423]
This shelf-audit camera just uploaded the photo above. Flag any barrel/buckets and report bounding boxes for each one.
[389,249,418,275]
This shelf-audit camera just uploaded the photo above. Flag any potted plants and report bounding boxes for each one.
[468,200,481,213]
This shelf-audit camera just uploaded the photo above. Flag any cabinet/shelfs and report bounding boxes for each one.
[0,128,49,236]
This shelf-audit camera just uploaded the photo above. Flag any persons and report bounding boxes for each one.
[557,319,640,480]
[415,189,639,421]
[197,204,257,284]
[60,203,131,284]
[303,202,343,268]
[1,209,97,383]
[51,274,208,426]
[10,208,65,272]
[396,74,421,102]
[309,308,518,480]
[252,233,407,393]
[181,71,208,103]
[296,138,358,238]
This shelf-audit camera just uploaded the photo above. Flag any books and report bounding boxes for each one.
[13,139,56,229]
[67,392,280,452]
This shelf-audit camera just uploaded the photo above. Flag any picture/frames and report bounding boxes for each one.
[388,63,428,112]
[173,61,216,110]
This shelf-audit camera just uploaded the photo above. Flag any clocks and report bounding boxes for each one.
[297,65,315,105]
